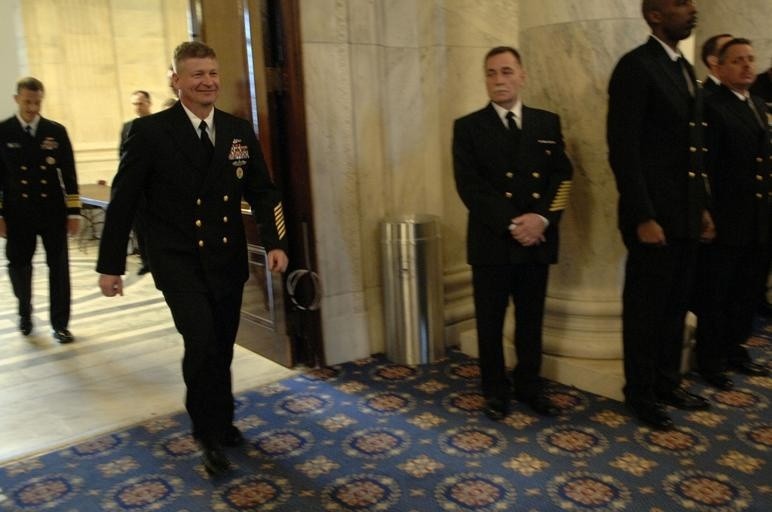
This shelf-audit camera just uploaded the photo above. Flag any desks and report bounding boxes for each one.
[76,184,138,256]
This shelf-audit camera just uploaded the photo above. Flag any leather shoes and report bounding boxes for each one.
[19,314,31,335]
[515,386,561,416]
[485,376,511,418]
[622,315,770,430]
[53,330,74,341]
[192,425,243,476]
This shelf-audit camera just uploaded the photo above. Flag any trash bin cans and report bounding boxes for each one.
[376,214,447,365]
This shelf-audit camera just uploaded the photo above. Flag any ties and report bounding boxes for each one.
[198,121,214,168]
[25,125,32,136]
[748,96,764,126]
[680,55,696,96]
[505,110,520,146]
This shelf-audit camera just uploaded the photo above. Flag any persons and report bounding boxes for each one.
[444,39,578,423]
[162,96,176,110]
[89,37,293,480]
[698,31,734,104]
[166,63,180,98]
[0,70,86,347]
[602,0,715,433]
[702,36,772,393]
[116,87,152,280]
